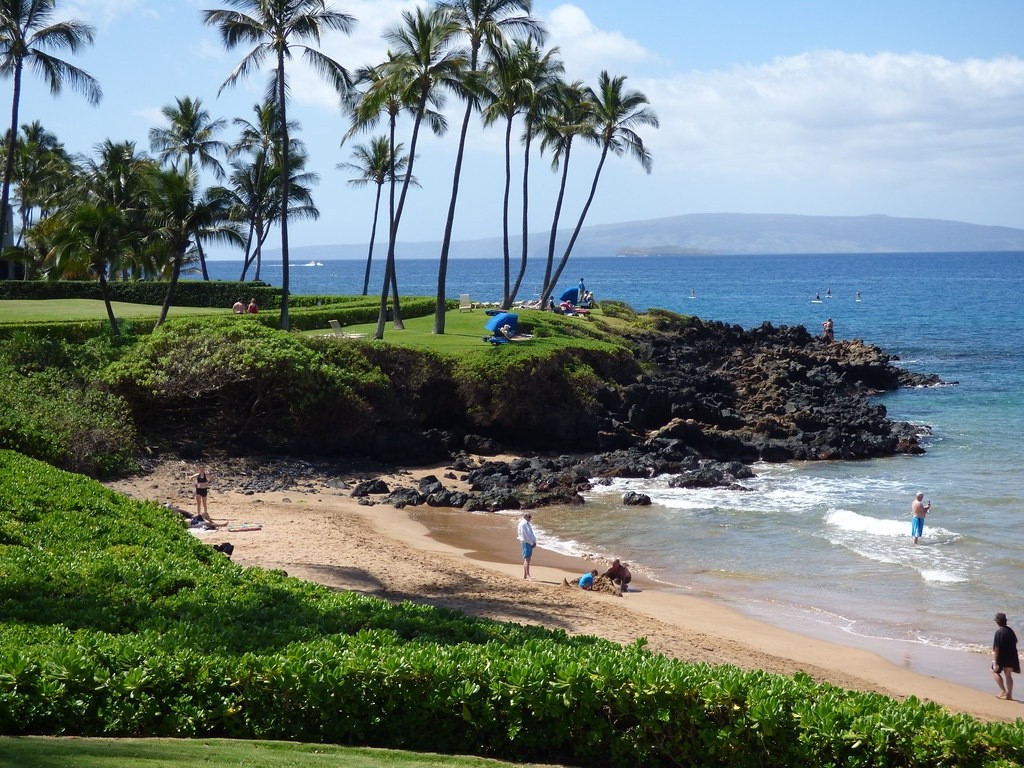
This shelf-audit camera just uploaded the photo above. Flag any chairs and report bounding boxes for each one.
[328,320,369,340]
[459,294,472,313]
[500,327,536,346]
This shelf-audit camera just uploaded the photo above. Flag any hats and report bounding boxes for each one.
[994,618,1007,622]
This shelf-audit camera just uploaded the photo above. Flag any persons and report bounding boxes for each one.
[518,512,536,582]
[233,297,247,314]
[827,287,831,297]
[691,287,695,297]
[566,300,589,313]
[248,298,258,313]
[911,492,930,544]
[578,277,599,308]
[547,296,556,309]
[823,318,834,341]
[600,558,631,593]
[179,512,229,528]
[816,290,819,301]
[190,466,212,515]
[856,290,861,300]
[991,613,1021,700]
[579,569,598,591]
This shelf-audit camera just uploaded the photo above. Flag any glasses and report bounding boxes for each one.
[526,516,531,518]
